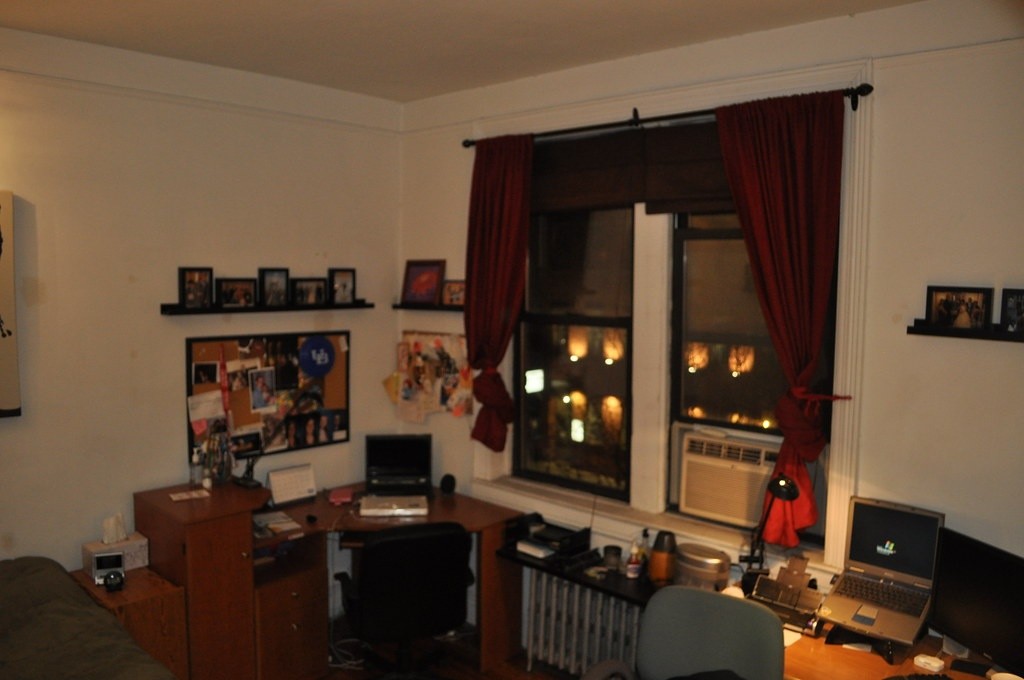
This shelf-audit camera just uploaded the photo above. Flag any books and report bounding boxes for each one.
[517,539,556,559]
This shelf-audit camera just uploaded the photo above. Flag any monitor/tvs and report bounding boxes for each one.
[927,524,1024,680]
[363,434,433,496]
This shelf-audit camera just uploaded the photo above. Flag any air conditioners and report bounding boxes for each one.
[671,421,831,540]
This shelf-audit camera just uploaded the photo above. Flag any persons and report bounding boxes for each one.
[184,275,351,307]
[935,292,983,328]
[196,349,344,454]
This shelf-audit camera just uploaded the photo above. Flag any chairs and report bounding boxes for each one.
[582,585,785,679]
[334,518,471,680]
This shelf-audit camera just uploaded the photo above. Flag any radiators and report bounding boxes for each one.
[522,565,640,680]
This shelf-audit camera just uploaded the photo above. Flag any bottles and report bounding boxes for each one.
[649,531,677,586]
[624,542,640,579]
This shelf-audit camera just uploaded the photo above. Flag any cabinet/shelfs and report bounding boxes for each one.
[132,476,524,680]
[72,568,187,679]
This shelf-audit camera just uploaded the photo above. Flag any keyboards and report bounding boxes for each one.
[359,494,428,516]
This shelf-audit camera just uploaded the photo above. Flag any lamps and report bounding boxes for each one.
[744,474,799,588]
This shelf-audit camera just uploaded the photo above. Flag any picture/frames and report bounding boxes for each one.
[175,266,356,308]
[186,329,357,465]
[1000,286,1024,332]
[400,260,445,303]
[924,285,995,330]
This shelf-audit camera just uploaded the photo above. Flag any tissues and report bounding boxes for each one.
[82,512,149,580]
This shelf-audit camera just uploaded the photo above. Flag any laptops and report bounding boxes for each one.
[816,495,946,645]
